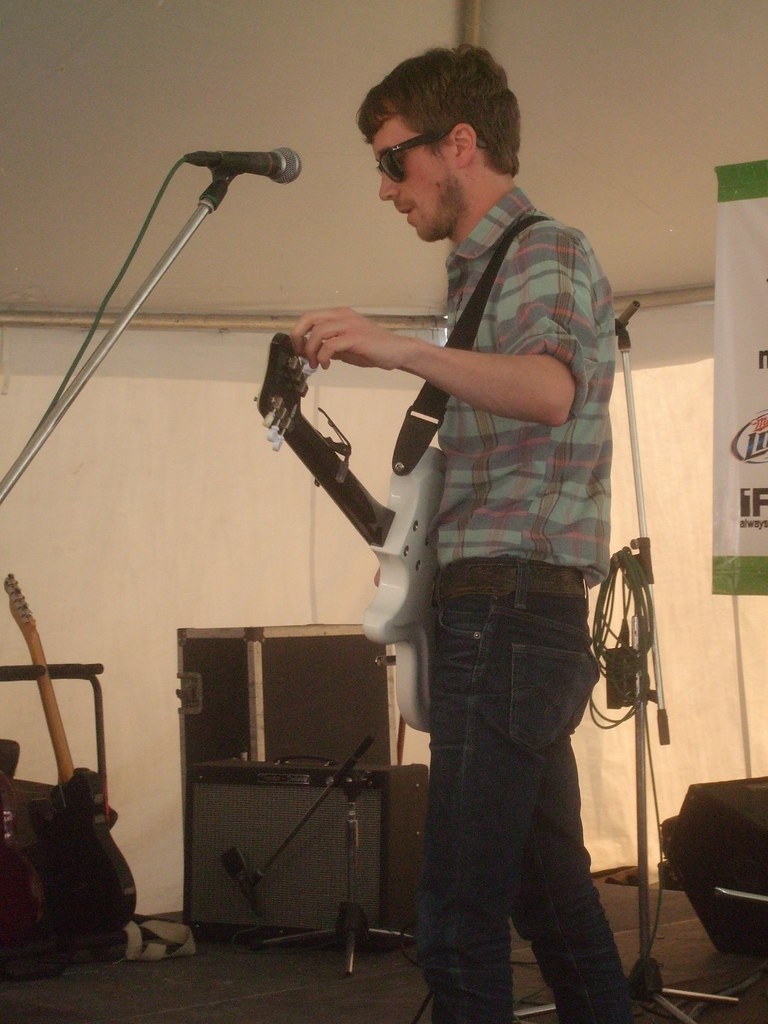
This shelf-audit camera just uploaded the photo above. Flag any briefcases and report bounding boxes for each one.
[182,754,429,946]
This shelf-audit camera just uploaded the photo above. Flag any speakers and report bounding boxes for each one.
[186,762,430,941]
[666,777,768,955]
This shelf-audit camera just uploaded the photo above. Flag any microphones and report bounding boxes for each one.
[183,147,301,185]
[221,847,261,913]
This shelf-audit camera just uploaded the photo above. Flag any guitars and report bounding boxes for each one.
[3,573,137,936]
[258,327,446,732]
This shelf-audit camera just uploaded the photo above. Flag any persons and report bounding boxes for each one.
[292,42,632,1024]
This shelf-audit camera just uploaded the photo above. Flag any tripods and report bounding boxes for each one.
[252,737,416,974]
[511,301,740,1024]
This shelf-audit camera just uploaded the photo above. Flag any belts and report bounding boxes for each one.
[434,560,587,600]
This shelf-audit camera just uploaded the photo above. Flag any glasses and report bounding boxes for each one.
[376,127,451,183]
